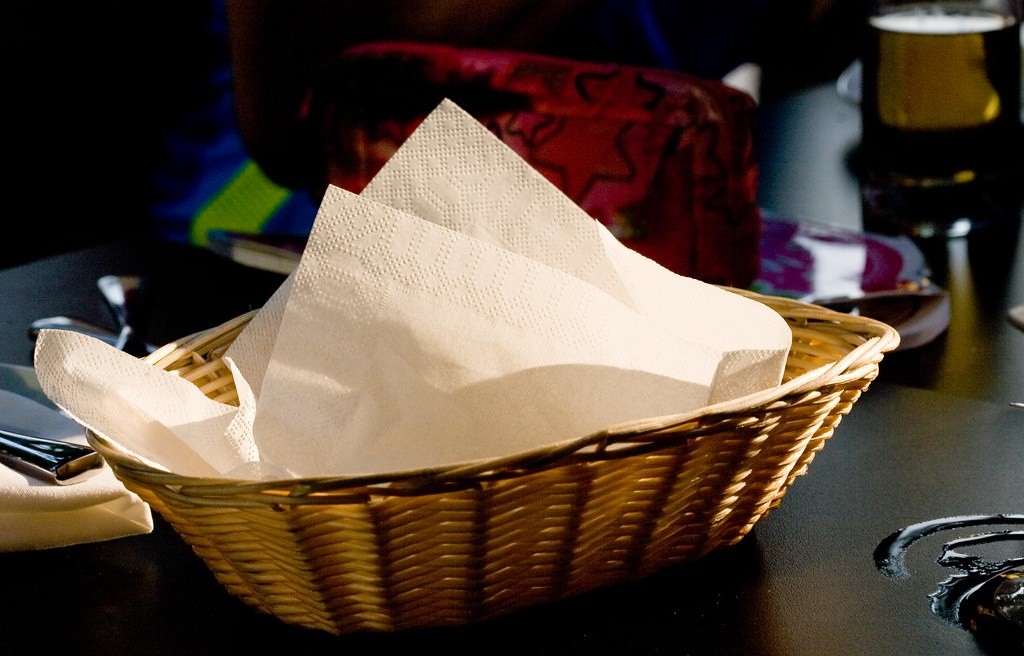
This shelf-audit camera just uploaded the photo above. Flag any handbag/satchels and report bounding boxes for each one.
[296,43,765,297]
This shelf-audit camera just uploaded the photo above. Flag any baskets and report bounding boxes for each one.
[86,289,901,642]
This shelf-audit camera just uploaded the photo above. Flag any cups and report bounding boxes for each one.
[868,0,1020,236]
